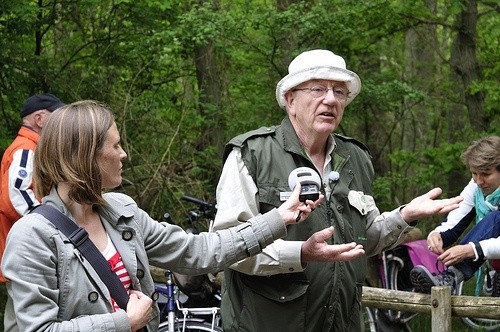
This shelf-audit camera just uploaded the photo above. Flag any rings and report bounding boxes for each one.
[427,246,433,251]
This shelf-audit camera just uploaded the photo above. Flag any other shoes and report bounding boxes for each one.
[409,264,455,295]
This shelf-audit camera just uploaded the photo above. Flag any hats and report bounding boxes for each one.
[20,93,66,116]
[276,49,362,111]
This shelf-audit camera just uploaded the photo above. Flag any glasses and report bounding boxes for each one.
[286,84,351,98]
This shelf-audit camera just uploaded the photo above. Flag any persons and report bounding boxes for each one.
[1,92,72,332]
[409,133,500,299]
[2,99,325,332]
[208,48,465,332]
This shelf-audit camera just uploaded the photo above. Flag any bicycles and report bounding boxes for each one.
[154,195,222,332]
[365,243,500,332]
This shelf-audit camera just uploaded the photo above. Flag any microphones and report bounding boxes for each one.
[329,171,340,182]
